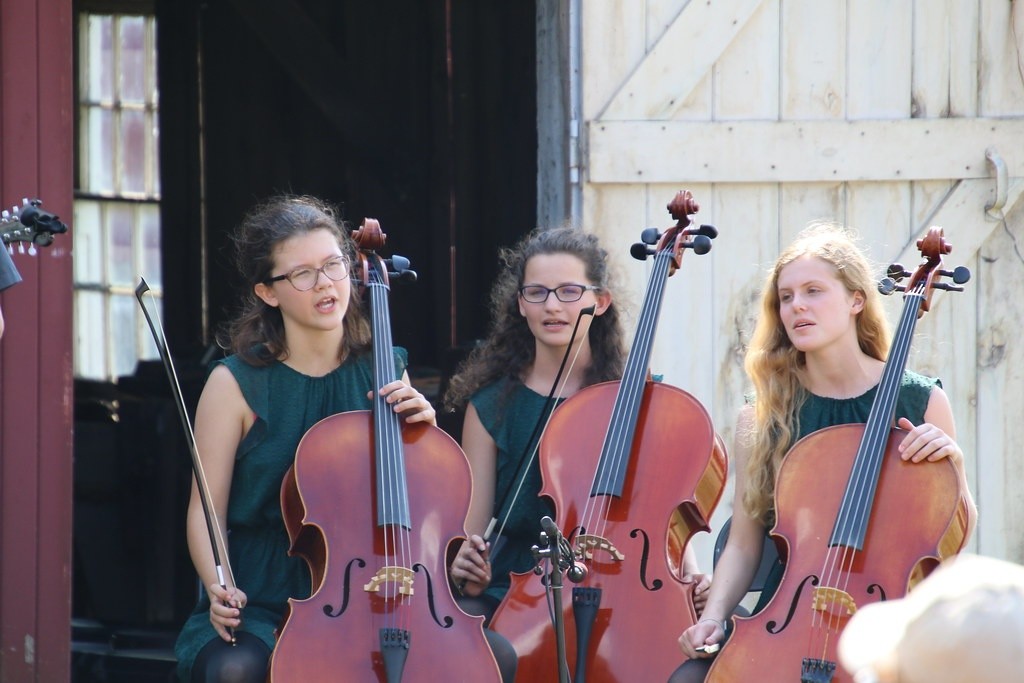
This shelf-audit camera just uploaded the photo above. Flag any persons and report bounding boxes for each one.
[443,228,711,626]
[669,234,979,683]
[175,199,518,683]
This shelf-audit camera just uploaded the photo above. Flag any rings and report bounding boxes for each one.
[694,646,704,651]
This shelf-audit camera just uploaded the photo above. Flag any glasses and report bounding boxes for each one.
[268,255,350,291]
[520,284,596,303]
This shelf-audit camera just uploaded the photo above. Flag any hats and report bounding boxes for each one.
[834,556,1024,683]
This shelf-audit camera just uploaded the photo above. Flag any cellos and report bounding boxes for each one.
[483,186,732,682]
[263,212,508,683]
[700,221,976,682]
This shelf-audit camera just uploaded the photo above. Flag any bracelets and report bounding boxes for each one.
[701,618,724,629]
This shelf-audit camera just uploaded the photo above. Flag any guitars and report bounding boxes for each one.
[0,194,71,258]
